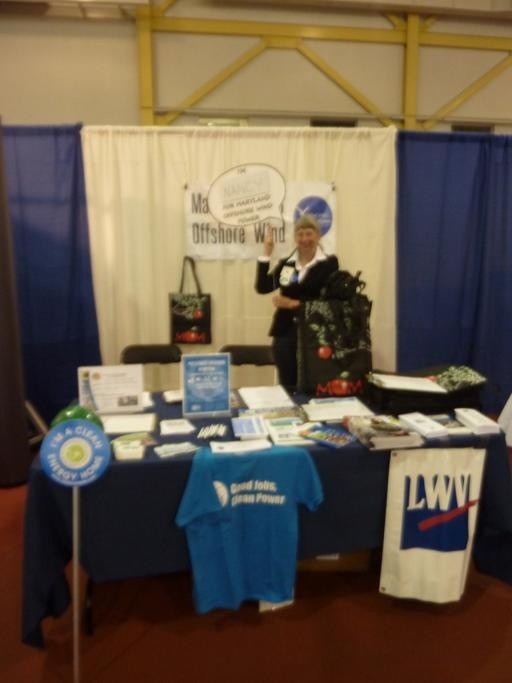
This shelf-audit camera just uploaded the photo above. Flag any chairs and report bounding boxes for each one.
[121,346,183,365]
[221,346,276,367]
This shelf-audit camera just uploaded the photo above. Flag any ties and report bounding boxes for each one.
[290,266,300,286]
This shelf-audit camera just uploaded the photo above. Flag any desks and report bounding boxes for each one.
[21,386,508,650]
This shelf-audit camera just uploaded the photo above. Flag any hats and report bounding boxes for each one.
[292,213,320,235]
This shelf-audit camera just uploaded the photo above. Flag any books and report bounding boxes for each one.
[342,414,421,451]
[264,416,318,447]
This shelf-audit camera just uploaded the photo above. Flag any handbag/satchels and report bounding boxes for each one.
[166,254,213,347]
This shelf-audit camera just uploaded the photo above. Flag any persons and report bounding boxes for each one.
[254,214,340,392]
